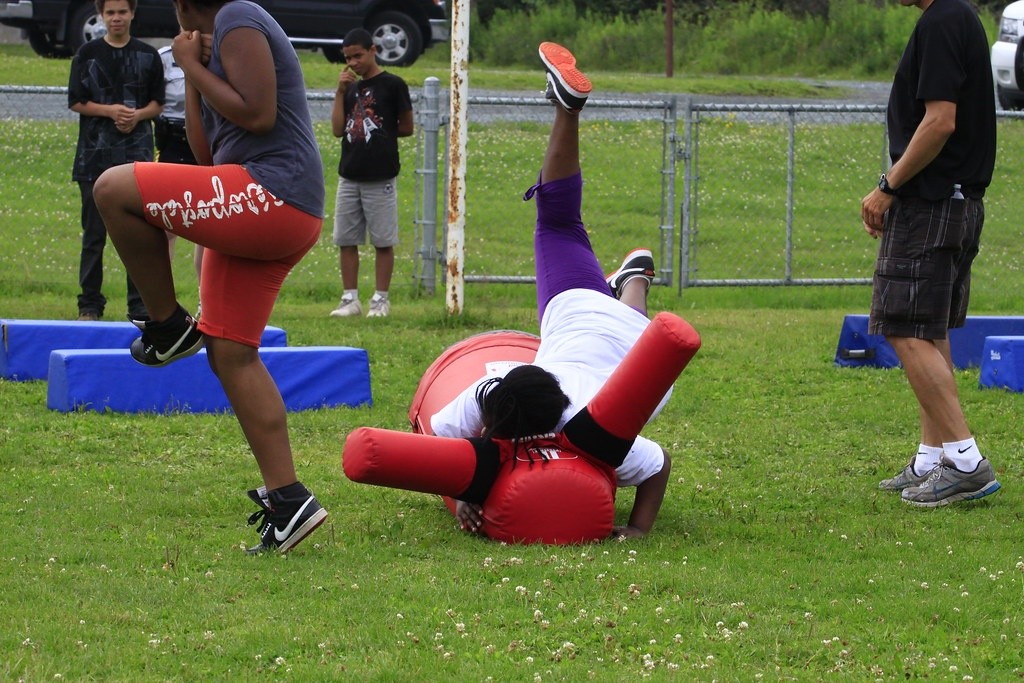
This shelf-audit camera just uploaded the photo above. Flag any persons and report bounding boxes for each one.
[154,45,204,321]
[69,1,166,321]
[93,0,329,560]
[428,42,674,545]
[863,0,1002,513]
[330,27,416,318]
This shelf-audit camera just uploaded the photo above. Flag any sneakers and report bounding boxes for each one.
[879,455,949,492]
[329,292,362,317]
[74,311,99,322]
[604,246,655,299]
[900,453,1001,507]
[242,479,329,554]
[126,302,205,366]
[538,40,592,114]
[366,294,390,318]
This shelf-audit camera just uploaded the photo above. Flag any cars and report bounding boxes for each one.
[0,0,447,69]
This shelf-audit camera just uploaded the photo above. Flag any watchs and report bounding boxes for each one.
[879,174,897,195]
